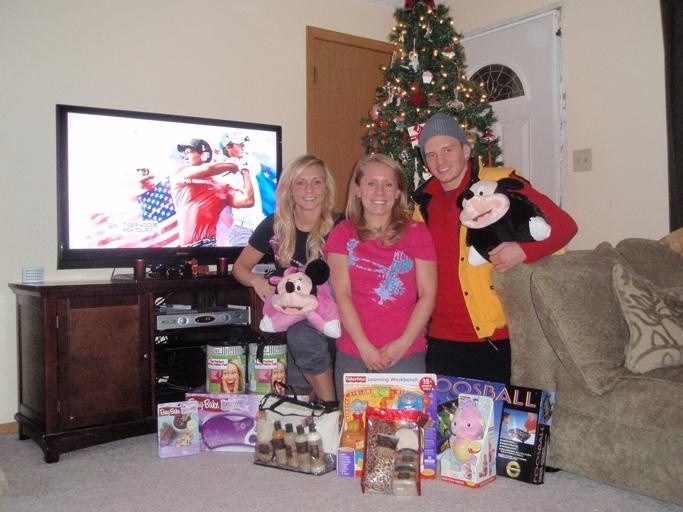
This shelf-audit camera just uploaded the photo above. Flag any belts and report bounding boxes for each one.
[191,239,216,247]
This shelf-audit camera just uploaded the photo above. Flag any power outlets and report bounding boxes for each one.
[570,147,593,174]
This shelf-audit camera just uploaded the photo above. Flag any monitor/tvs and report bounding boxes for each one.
[55,103,283,280]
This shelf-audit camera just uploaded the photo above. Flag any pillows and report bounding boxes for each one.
[612,258,683,375]
[530,239,635,399]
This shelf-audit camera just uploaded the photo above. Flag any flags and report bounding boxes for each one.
[80,176,180,246]
[257,158,276,216]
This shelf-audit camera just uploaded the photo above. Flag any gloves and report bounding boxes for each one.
[237,158,249,175]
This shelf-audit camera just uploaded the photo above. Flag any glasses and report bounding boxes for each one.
[180,150,196,160]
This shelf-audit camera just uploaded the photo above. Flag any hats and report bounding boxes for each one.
[219,134,245,149]
[136,168,149,175]
[176,138,212,162]
[418,113,466,171]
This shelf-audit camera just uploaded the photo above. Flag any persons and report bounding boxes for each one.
[269,363,286,396]
[410,111,579,390]
[132,165,152,190]
[500,408,537,445]
[218,360,241,394]
[172,139,255,248]
[230,157,345,403]
[213,132,267,246]
[321,153,440,383]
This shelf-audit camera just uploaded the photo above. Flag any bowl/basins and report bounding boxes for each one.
[172,414,192,434]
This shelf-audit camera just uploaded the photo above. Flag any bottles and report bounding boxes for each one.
[340,420,365,449]
[253,409,325,475]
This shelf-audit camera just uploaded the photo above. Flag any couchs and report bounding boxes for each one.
[489,225,683,508]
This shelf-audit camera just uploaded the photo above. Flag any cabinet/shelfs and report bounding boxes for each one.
[147,279,255,437]
[7,277,149,464]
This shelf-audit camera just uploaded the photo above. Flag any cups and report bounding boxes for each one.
[132,258,147,278]
[216,257,228,274]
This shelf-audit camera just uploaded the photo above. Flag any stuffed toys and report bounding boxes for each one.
[256,257,342,340]
[448,400,490,476]
[450,179,567,266]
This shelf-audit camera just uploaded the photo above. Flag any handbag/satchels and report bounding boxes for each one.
[252,381,343,476]
